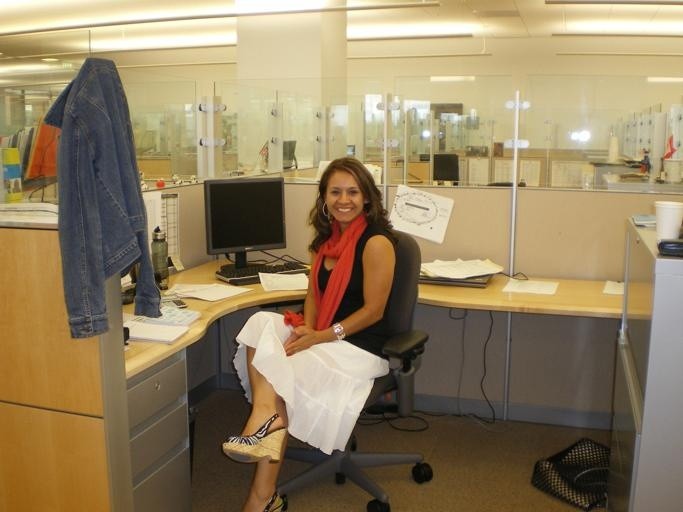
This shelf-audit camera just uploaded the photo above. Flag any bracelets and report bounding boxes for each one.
[331,321,345,340]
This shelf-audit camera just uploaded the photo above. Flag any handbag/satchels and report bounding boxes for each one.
[531,437,611,511]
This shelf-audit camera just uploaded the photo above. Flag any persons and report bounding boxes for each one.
[222,156,399,511]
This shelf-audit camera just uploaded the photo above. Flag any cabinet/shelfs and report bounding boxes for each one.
[125,356,194,511]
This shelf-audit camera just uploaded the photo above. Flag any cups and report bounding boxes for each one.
[654,200,682,242]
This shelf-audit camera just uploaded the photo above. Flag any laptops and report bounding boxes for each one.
[418,272,493,289]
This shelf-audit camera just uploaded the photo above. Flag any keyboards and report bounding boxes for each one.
[214,261,308,285]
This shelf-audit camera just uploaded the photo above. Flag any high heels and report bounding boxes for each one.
[260,490,288,511]
[223,414,287,464]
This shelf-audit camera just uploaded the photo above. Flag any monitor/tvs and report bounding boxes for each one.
[419,153,460,182]
[204,177,286,271]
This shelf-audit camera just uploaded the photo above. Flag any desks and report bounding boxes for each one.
[124,254,656,379]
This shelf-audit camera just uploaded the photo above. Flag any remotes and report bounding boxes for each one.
[173,299,187,308]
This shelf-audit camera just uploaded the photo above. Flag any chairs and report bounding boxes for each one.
[271,228,435,512]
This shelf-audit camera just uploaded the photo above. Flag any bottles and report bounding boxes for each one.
[151,231,170,290]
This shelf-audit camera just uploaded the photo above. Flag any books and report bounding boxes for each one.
[123,319,188,343]
[418,274,493,289]
